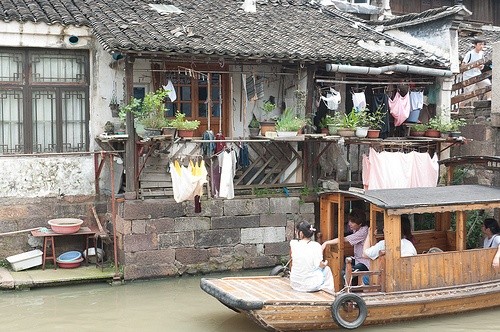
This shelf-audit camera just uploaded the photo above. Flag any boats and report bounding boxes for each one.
[200,153,500,332]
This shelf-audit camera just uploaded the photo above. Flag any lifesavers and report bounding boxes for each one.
[269,265,290,276]
[330,293,368,329]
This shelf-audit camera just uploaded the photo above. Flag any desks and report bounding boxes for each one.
[31,227,103,270]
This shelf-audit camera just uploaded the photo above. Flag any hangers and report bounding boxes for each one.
[319,79,425,92]
[208,141,244,159]
[167,139,203,160]
[175,62,209,81]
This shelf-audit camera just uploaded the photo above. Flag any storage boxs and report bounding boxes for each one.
[6,249,43,272]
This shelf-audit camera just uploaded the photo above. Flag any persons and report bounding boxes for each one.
[481,218,500,269]
[462,39,491,107]
[321,210,418,293]
[289,221,335,293]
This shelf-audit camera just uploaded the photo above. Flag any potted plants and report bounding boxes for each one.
[247,100,317,138]
[109,88,201,138]
[411,115,467,137]
[319,103,388,138]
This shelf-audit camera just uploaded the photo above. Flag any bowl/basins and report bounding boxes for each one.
[83,247,105,264]
[48,218,84,234]
[56,257,84,268]
[59,251,81,260]
